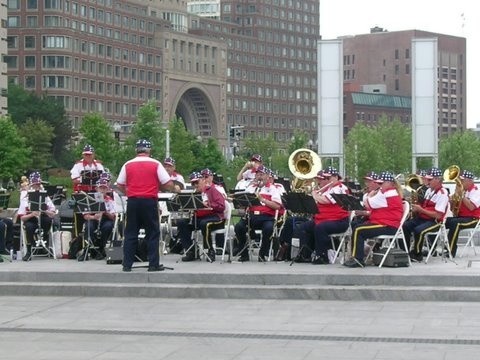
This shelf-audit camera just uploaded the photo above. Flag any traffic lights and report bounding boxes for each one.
[230,129,234,137]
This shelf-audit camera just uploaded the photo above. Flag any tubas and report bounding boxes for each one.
[405,173,424,220]
[288,147,323,192]
[443,164,465,217]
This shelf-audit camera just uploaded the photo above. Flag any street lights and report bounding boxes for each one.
[113,121,121,170]
[233,142,237,158]
[309,139,313,150]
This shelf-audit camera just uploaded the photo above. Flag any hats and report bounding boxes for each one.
[189,172,203,181]
[418,167,443,179]
[321,166,338,177]
[255,165,278,180]
[459,170,475,180]
[164,157,175,167]
[136,137,151,148]
[96,173,112,188]
[200,168,214,176]
[361,171,381,181]
[249,154,262,162]
[374,171,395,184]
[314,170,325,179]
[82,144,95,155]
[28,171,49,189]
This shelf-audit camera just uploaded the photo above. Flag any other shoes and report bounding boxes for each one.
[409,250,423,262]
[95,248,106,260]
[22,252,31,261]
[291,253,329,263]
[123,266,131,271]
[78,252,88,261]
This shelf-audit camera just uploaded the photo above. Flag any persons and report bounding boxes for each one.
[116,139,181,271]
[178,171,225,262]
[68,178,116,260]
[0,172,56,264]
[63,143,480,268]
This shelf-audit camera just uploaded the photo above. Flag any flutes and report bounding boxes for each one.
[241,180,261,221]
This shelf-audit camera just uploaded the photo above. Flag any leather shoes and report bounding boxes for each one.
[182,245,201,260]
[343,258,367,268]
[237,249,267,261]
[148,266,165,271]
[207,248,215,261]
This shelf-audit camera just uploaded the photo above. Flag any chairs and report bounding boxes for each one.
[9,198,480,268]
